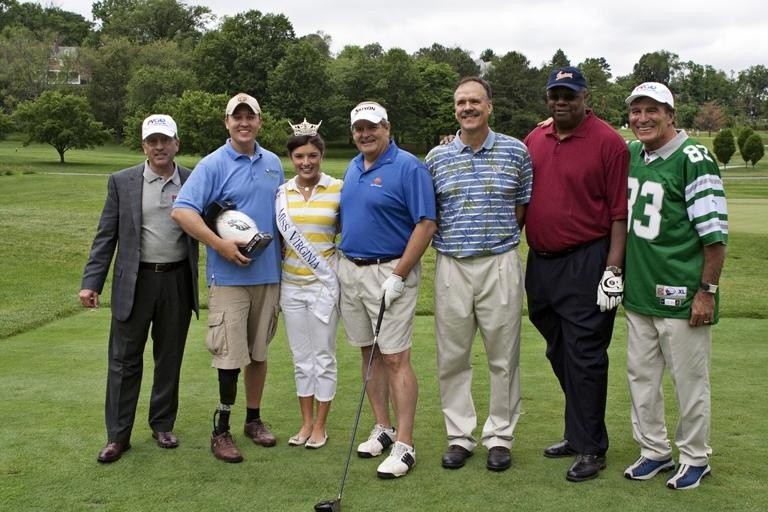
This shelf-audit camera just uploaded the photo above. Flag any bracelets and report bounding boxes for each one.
[696,279,720,293]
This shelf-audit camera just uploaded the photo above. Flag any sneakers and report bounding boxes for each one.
[358,425,397,457]
[666,464,710,490]
[624,456,675,481]
[377,442,415,478]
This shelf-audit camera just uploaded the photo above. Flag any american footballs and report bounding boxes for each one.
[216,210,259,247]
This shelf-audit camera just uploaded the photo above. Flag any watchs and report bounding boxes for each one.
[603,264,625,276]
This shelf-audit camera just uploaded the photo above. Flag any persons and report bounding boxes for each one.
[421,77,534,470]
[169,92,286,463]
[281,103,438,479]
[536,81,729,490]
[80,114,199,463]
[437,67,627,480]
[270,130,344,449]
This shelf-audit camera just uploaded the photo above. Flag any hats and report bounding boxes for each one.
[624,82,674,109]
[350,101,388,129]
[142,114,179,140]
[226,93,261,118]
[547,68,587,91]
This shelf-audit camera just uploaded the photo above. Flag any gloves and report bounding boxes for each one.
[595,266,625,311]
[377,275,404,309]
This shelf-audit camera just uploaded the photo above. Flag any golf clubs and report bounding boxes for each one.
[314,294,385,512]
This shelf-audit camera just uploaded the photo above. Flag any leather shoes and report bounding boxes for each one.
[151,431,179,448]
[566,453,606,481]
[288,432,328,448]
[244,418,276,447]
[543,440,578,457]
[442,445,472,468]
[211,429,242,462]
[97,441,131,461]
[486,447,511,470]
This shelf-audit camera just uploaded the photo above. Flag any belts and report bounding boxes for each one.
[344,253,403,266]
[138,261,186,271]
[529,242,590,258]
